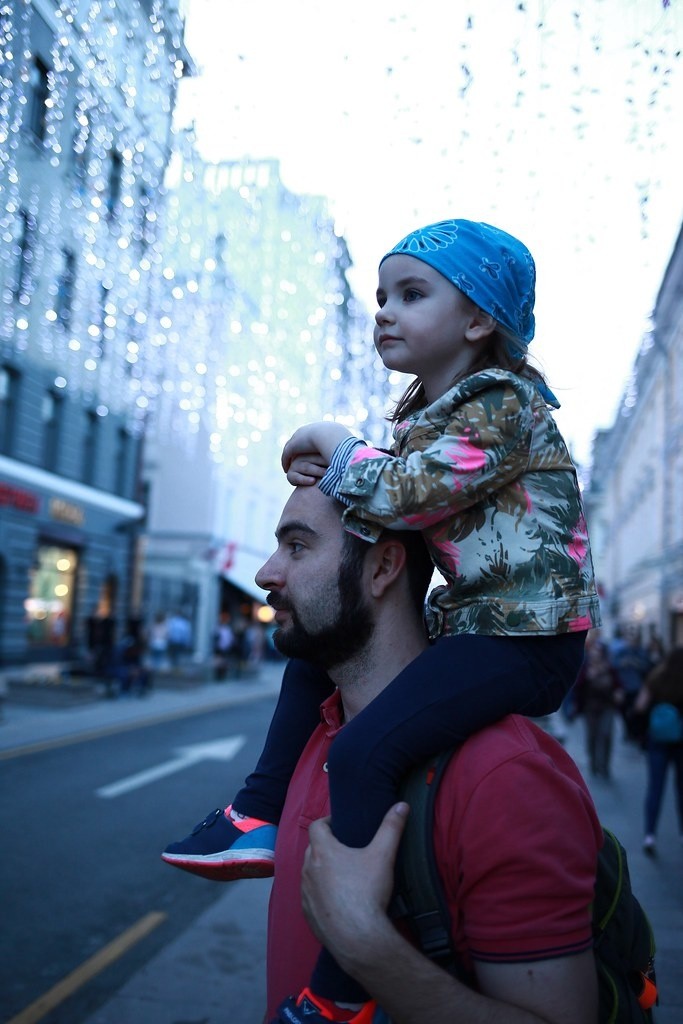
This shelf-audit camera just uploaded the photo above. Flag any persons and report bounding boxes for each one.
[254,447,610,1024]
[543,607,683,855]
[87,604,263,701]
[161,219,601,1024]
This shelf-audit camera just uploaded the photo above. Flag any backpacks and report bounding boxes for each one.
[404,728,659,1024]
[652,701,681,743]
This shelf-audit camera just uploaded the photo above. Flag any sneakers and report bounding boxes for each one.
[270,986,382,1024]
[161,808,277,879]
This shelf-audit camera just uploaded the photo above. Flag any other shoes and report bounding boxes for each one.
[642,837,657,852]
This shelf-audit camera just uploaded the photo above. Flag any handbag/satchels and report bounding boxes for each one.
[561,677,581,722]
